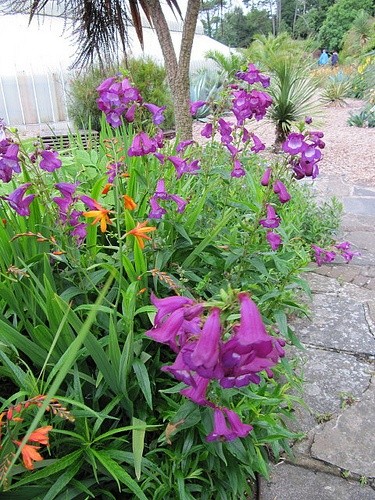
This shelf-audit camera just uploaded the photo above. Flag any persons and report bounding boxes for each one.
[318,49,328,66]
[330,50,339,67]
